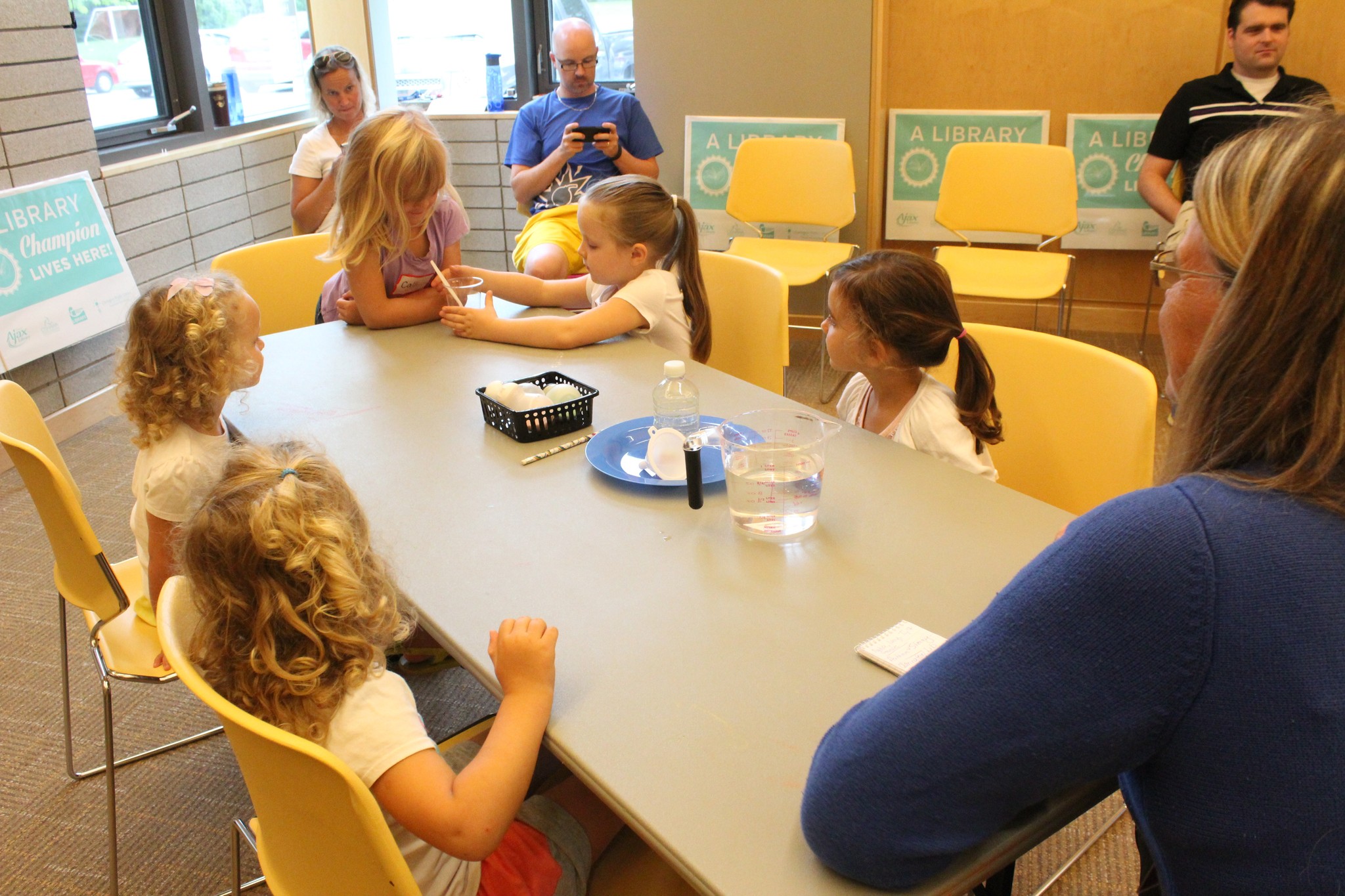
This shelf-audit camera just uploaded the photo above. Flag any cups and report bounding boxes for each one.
[207,82,230,127]
[442,277,484,330]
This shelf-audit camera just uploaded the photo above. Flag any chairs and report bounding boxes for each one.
[0,375,473,896]
[210,234,339,340]
[680,137,1187,515]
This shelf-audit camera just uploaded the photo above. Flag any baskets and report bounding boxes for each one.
[475,371,599,443]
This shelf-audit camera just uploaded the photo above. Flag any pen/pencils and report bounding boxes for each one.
[521,429,604,465]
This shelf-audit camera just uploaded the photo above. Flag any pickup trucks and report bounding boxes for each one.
[70,4,153,96]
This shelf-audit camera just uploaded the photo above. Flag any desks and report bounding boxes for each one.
[221,288,1139,896]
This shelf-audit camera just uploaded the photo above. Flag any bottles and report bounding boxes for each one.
[485,53,504,112]
[652,360,700,439]
[221,68,244,126]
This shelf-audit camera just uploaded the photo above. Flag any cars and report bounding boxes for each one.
[199,12,316,91]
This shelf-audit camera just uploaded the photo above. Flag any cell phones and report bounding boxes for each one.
[572,126,611,142]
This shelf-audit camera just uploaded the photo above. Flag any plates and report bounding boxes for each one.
[585,415,766,486]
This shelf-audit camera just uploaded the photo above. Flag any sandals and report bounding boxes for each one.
[385,642,460,680]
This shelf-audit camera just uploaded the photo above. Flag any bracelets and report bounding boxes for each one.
[609,142,622,161]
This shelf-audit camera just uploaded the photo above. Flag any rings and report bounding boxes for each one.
[607,141,609,147]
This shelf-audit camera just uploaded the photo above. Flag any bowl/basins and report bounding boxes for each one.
[398,99,431,112]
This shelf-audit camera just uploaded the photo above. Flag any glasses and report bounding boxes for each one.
[312,51,354,88]
[1149,250,1236,293]
[554,53,598,71]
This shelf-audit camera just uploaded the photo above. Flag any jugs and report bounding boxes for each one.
[682,409,843,536]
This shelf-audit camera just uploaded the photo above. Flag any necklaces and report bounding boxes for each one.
[557,83,597,111]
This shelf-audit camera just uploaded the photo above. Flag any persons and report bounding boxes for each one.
[1137,0,1336,250]
[107,274,457,675]
[821,250,1006,484]
[289,45,377,234]
[168,436,705,896]
[315,109,471,329]
[801,113,1345,896]
[502,19,665,279]
[431,174,712,364]
